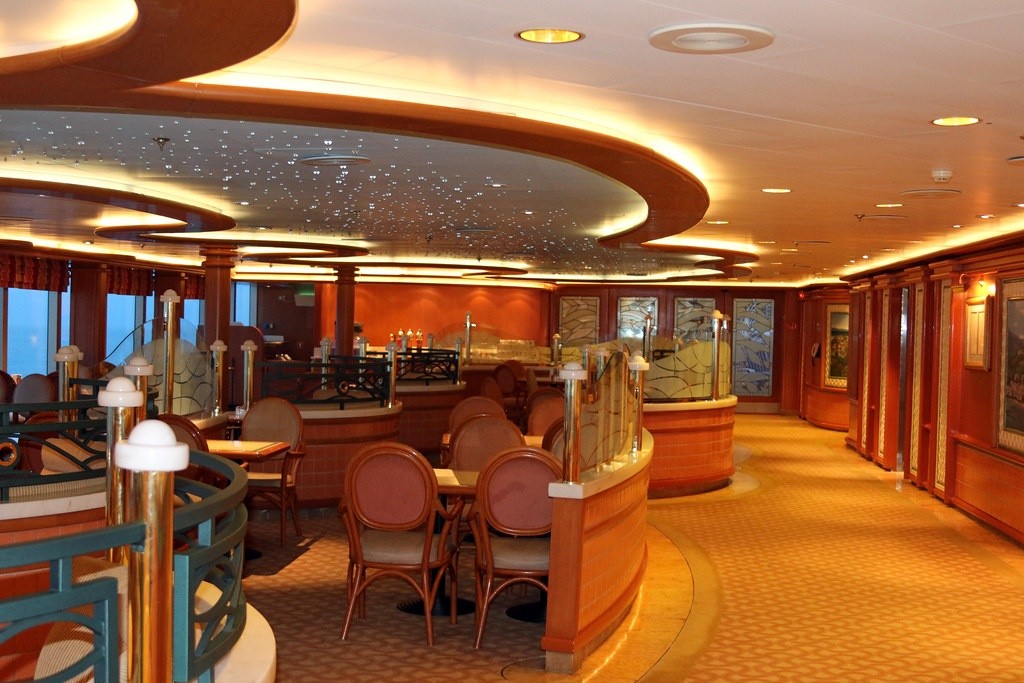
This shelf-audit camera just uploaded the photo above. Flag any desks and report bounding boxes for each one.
[205,438,291,562]
[428,467,480,631]
[442,433,544,448]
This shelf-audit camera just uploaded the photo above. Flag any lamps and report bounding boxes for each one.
[959,273,985,293]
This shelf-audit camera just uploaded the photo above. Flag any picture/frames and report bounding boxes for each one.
[996,276,1024,457]
[823,302,851,388]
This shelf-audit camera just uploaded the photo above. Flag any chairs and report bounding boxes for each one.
[155,359,566,647]
[0,369,61,477]
